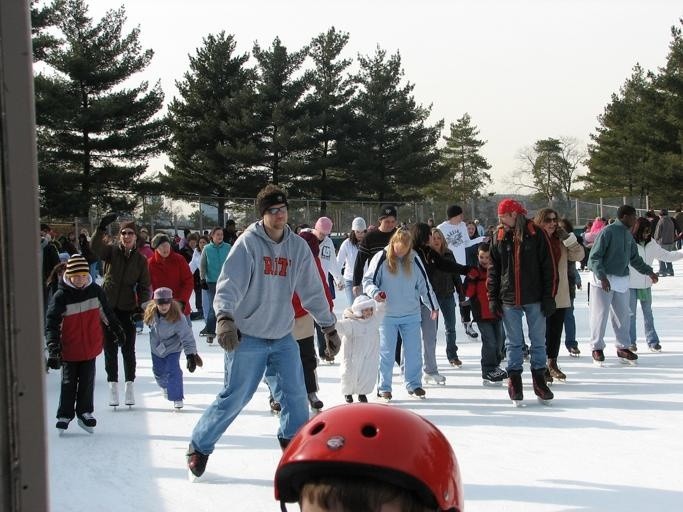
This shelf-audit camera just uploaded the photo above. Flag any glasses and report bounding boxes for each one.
[120,230,134,237]
[546,217,561,224]
[267,205,288,214]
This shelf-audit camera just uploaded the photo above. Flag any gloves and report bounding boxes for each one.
[540,296,555,318]
[322,325,342,357]
[108,320,127,346]
[487,299,504,319]
[184,353,197,373]
[214,312,242,353]
[199,278,209,291]
[47,345,65,370]
[553,224,569,241]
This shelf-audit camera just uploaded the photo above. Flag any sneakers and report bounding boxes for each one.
[424,373,446,385]
[358,395,368,403]
[628,346,637,353]
[508,369,523,407]
[307,392,324,412]
[649,344,662,353]
[408,387,425,400]
[344,395,353,403]
[531,368,554,406]
[617,346,638,366]
[567,346,581,357]
[448,356,462,368]
[188,442,209,478]
[174,400,185,409]
[379,392,392,400]
[482,370,503,387]
[76,412,97,428]
[548,360,566,383]
[495,367,508,383]
[592,350,605,367]
[544,366,553,386]
[55,417,72,430]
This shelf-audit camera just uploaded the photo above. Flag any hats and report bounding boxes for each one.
[378,205,398,223]
[119,220,137,232]
[315,217,333,235]
[153,286,173,305]
[64,253,90,278]
[256,184,290,216]
[351,294,377,317]
[351,217,366,231]
[150,233,171,249]
[446,205,463,220]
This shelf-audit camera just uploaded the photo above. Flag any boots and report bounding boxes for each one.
[108,381,121,406]
[464,320,479,339]
[124,381,136,406]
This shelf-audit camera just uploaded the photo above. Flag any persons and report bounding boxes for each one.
[40,205,238,433]
[184,182,683,477]
